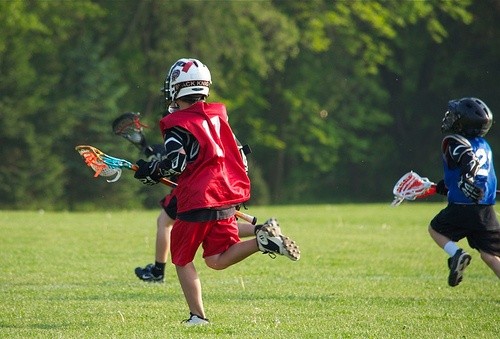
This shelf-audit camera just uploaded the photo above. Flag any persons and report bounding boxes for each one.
[426,96,500,287]
[132,100,281,285]
[133,58,301,328]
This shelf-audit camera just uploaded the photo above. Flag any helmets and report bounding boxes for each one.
[161,57,212,100]
[441,97,494,136]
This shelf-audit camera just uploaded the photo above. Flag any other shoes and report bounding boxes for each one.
[264,218,282,233]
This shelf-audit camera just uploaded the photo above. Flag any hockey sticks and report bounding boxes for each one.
[111,111,174,182]
[75,145,259,225]
[392,170,500,203]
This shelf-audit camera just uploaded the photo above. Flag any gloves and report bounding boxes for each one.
[134,158,169,186]
[456,171,483,203]
[435,179,449,196]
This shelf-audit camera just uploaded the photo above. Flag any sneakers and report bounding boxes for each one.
[135,263,165,282]
[256,223,301,261]
[181,312,210,324]
[447,248,473,287]
